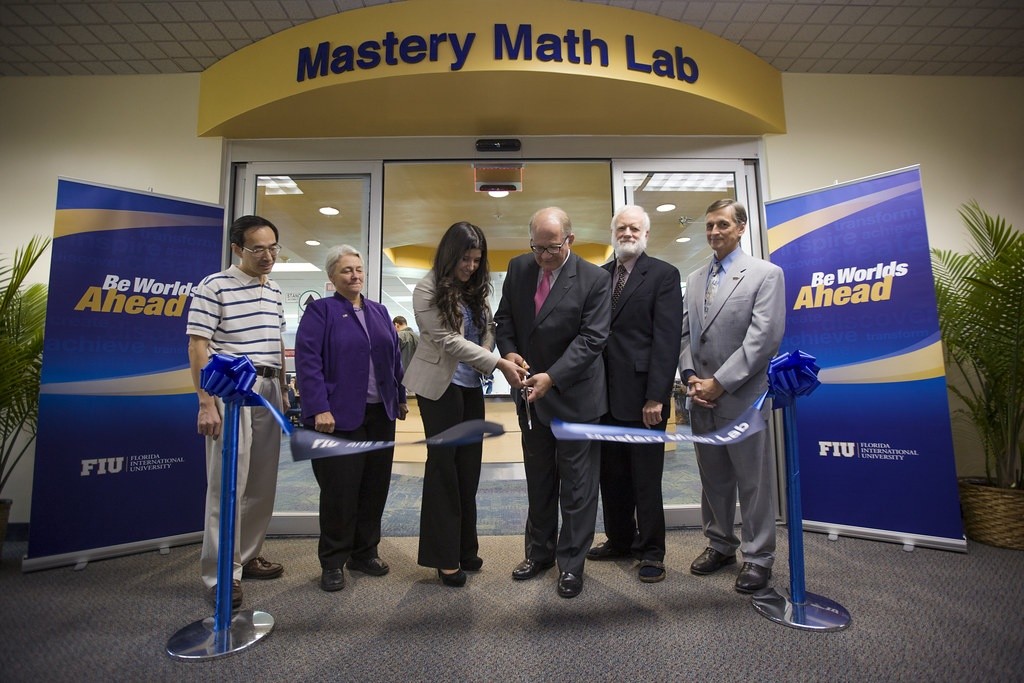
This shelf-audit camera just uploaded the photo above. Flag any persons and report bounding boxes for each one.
[295,245,408,592]
[400,221,530,587]
[186,215,291,610]
[393,316,420,373]
[586,206,683,583]
[678,199,786,594]
[494,207,612,598]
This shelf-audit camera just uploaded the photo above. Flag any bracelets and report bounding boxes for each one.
[280,384,289,391]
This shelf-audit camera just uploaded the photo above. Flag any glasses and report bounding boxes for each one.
[243,244,281,258]
[530,235,569,254]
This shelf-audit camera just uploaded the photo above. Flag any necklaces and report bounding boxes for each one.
[355,299,363,312]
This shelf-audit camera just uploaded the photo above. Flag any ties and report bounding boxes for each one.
[705,264,722,322]
[534,270,552,318]
[611,265,627,312]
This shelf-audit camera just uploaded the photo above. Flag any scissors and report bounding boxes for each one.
[521,359,532,430]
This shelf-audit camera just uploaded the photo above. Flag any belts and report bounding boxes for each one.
[255,366,280,379]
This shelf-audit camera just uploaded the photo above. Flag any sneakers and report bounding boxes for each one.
[242,556,284,580]
[586,540,640,561]
[639,556,665,583]
[212,582,243,609]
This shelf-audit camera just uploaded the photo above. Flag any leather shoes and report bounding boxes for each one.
[320,564,345,591]
[690,547,736,575]
[346,556,390,576]
[735,562,772,594]
[558,571,582,596]
[512,557,556,579]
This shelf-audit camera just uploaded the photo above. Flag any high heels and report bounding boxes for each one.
[460,557,483,571]
[437,568,466,587]
